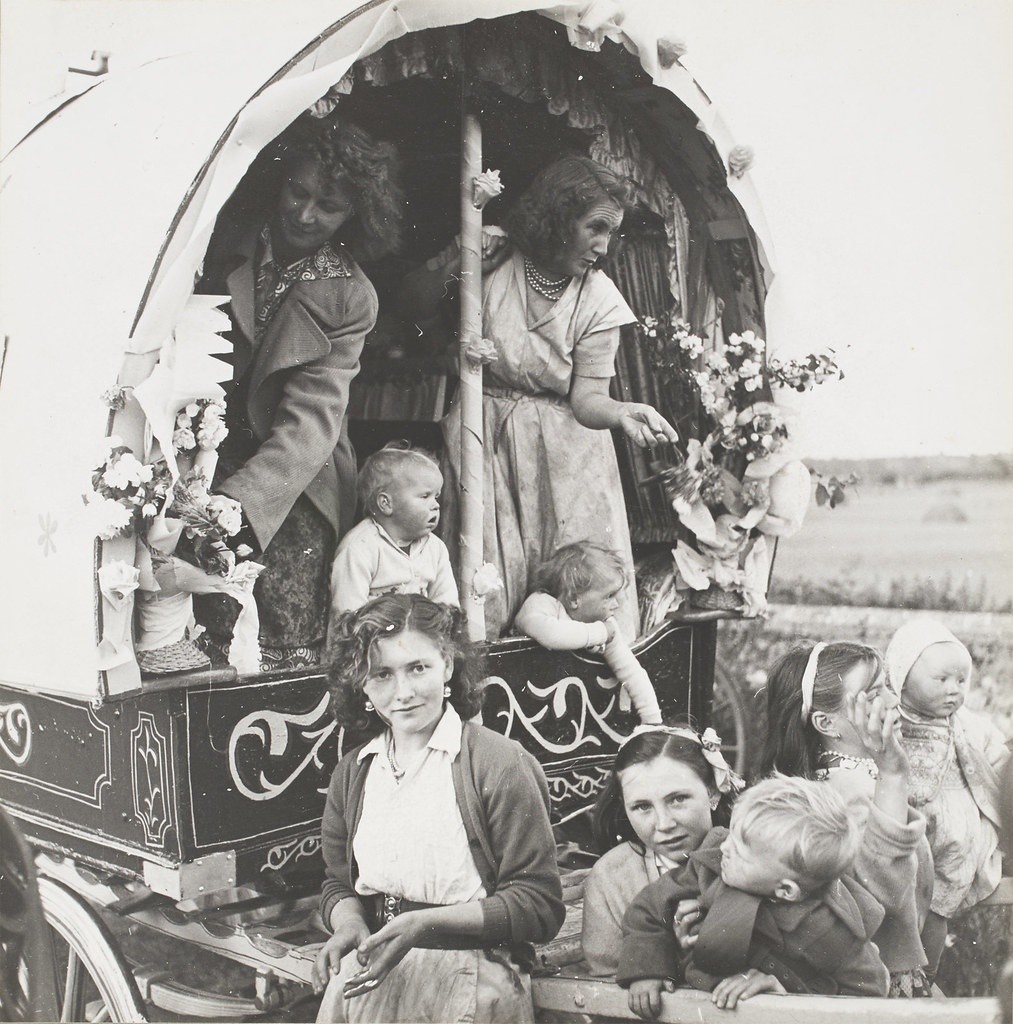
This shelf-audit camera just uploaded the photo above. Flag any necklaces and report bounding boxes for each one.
[523,256,570,301]
[816,751,875,766]
[387,736,406,780]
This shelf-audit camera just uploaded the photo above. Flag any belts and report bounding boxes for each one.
[365,893,479,950]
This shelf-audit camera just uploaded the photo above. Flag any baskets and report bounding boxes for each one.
[137,537,211,672]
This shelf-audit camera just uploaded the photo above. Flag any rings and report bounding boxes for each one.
[674,915,681,925]
[739,972,749,980]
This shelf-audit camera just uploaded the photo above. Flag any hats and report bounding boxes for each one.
[886,619,971,703]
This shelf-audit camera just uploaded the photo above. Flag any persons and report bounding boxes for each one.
[760,640,937,997]
[307,594,567,1024]
[579,713,791,1012]
[194,119,405,650]
[401,152,679,640]
[509,534,663,727]
[321,439,461,668]
[883,618,1013,986]
[617,759,887,1020]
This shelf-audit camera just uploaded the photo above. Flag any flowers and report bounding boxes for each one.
[472,169,505,206]
[636,311,865,510]
[82,382,254,573]
[731,144,756,180]
[465,338,500,374]
